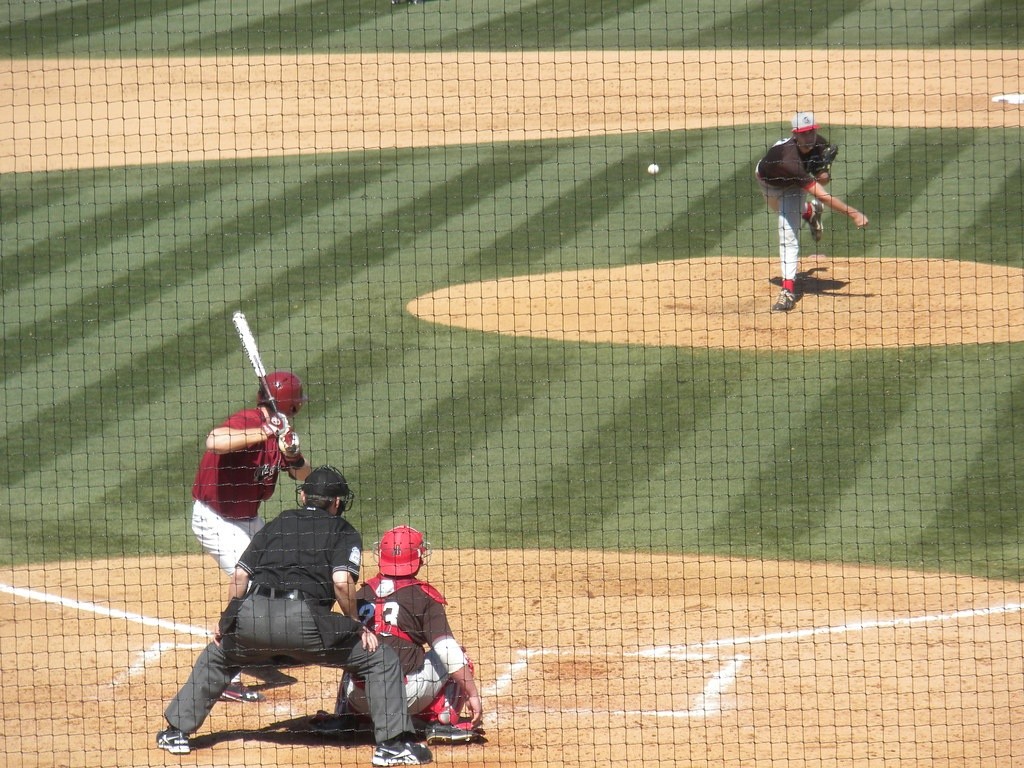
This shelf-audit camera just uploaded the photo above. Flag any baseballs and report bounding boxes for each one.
[647,164,659,175]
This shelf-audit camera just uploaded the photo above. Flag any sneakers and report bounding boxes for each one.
[770,289,799,312]
[244,666,296,684]
[157,727,191,754]
[220,677,264,702]
[810,200,825,241]
[372,741,433,767]
[424,723,483,741]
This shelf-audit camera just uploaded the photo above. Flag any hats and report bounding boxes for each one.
[791,111,819,134]
[303,465,349,497]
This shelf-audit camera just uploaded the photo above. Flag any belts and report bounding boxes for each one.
[251,584,303,602]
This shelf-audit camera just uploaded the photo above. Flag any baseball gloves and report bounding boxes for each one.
[807,142,839,174]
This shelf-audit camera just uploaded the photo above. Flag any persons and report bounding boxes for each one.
[192,371,312,703]
[156,465,433,766]
[345,523,482,746]
[755,115,868,312]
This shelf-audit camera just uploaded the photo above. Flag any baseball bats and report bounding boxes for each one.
[232,309,295,455]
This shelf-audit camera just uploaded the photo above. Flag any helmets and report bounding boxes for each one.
[258,372,306,417]
[379,525,424,577]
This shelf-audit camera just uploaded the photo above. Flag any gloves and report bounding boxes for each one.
[261,410,289,438]
[279,429,300,462]
